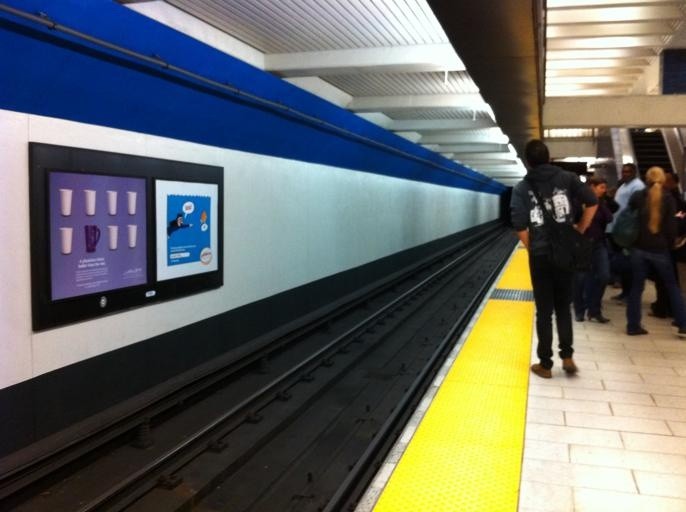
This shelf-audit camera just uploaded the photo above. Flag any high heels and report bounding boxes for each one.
[588,310,611,324]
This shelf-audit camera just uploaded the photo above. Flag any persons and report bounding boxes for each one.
[510,140,599,378]
[572,163,686,340]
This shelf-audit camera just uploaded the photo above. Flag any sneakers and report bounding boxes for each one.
[562,358,577,375]
[610,292,629,302]
[575,310,586,321]
[626,325,648,336]
[531,363,552,378]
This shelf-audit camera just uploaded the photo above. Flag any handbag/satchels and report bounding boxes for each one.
[547,222,593,275]
